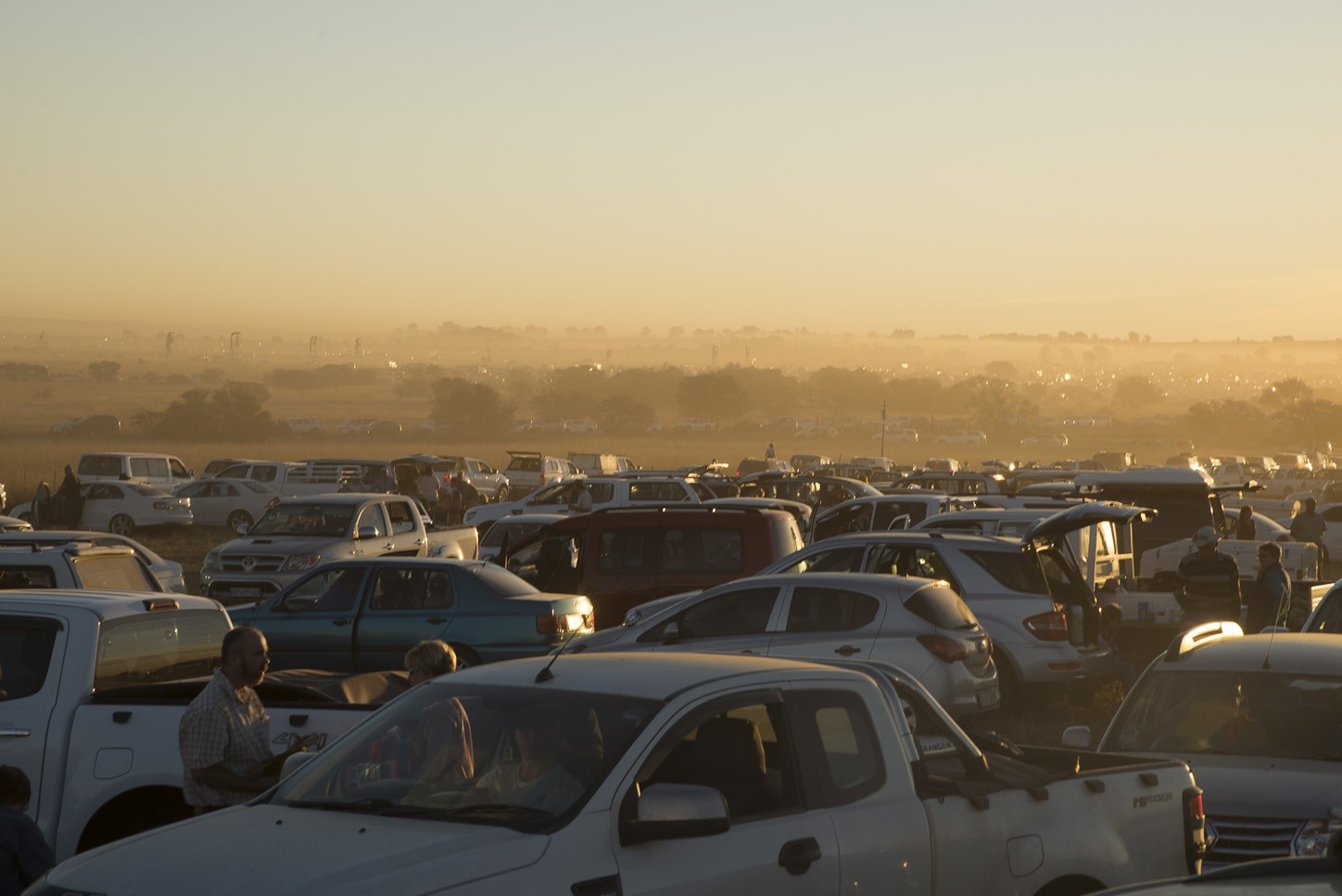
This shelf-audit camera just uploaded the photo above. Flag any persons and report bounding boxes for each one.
[367,467,490,526]
[0,764,53,896]
[397,640,475,796]
[1245,542,1291,634]
[1173,526,1241,622]
[764,442,775,460]
[476,707,586,816]
[533,539,577,592]
[54,464,81,530]
[1227,505,1255,540]
[1102,604,1136,695]
[1290,497,1328,581]
[118,473,131,481]
[178,627,308,819]
[568,479,593,515]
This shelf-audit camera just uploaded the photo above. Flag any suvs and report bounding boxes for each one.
[1091,618,1342,865]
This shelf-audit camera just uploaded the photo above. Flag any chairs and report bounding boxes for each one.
[673,716,784,824]
[254,471,262,482]
[405,569,426,610]
[371,568,405,611]
[266,470,275,482]
[799,485,814,506]
[228,485,237,496]
[660,488,682,500]
[547,698,604,792]
[213,486,222,497]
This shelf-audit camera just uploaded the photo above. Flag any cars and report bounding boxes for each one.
[222,553,598,677]
[0,443,1342,896]
[7,478,194,539]
[545,568,1001,751]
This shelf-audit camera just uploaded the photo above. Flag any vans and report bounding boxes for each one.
[74,450,195,497]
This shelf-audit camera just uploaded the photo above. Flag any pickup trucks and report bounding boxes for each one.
[17,647,1221,896]
[195,491,482,607]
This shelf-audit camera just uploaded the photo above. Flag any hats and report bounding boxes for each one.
[1191,525,1222,547]
